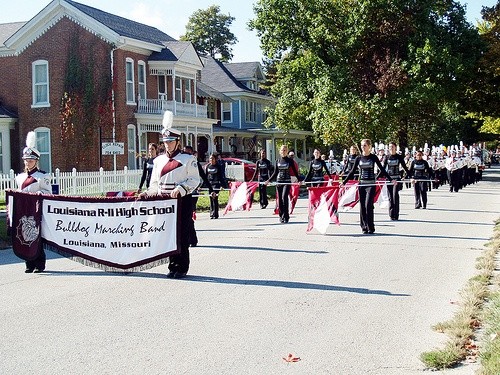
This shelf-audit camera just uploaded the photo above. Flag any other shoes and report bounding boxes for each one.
[261,204,268,209]
[362,230,374,234]
[25,268,43,273]
[415,206,426,209]
[210,216,218,219]
[281,218,289,223]
[392,217,398,220]
[167,271,186,278]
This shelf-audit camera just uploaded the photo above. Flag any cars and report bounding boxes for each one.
[323,160,343,175]
[482,149,500,168]
[202,158,258,181]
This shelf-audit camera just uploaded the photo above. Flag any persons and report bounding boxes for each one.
[264,144,302,223]
[181,145,226,247]
[375,141,415,221]
[249,137,500,210]
[7,147,52,273]
[138,142,167,194]
[339,139,398,235]
[233,133,239,157]
[138,128,201,279]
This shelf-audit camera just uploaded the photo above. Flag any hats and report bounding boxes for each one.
[21,147,40,169]
[162,128,181,152]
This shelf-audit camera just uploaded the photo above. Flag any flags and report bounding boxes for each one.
[247,134,258,159]
[223,175,387,235]
